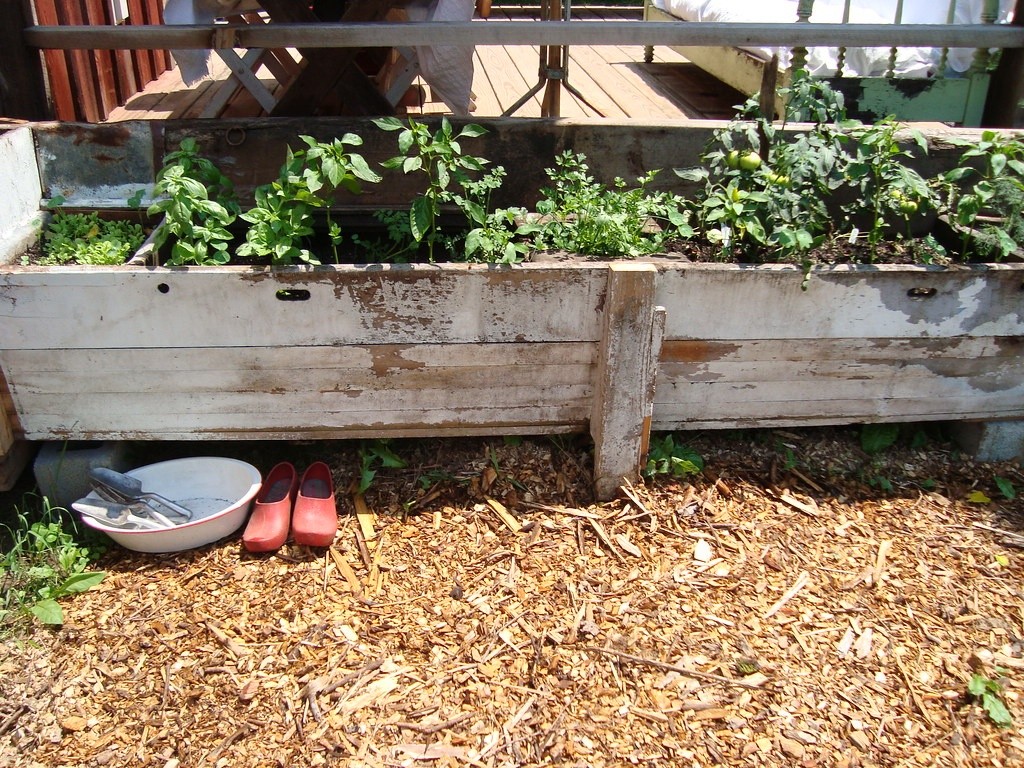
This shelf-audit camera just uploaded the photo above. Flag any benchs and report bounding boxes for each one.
[639,0,1023,129]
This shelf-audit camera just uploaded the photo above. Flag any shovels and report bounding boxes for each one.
[71,498,168,530]
[89,479,176,528]
[87,467,193,520]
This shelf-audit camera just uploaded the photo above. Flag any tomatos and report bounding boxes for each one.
[888,189,918,213]
[728,149,790,200]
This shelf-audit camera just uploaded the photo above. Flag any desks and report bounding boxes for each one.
[258,0,402,118]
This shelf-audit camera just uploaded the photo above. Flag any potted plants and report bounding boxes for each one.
[0,62,1024,496]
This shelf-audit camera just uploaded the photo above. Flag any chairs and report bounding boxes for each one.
[385,0,479,115]
[164,0,296,119]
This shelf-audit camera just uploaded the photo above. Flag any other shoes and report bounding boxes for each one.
[292,461,337,546]
[243,462,299,554]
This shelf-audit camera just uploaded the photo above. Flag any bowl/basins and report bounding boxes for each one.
[79,456,263,552]
